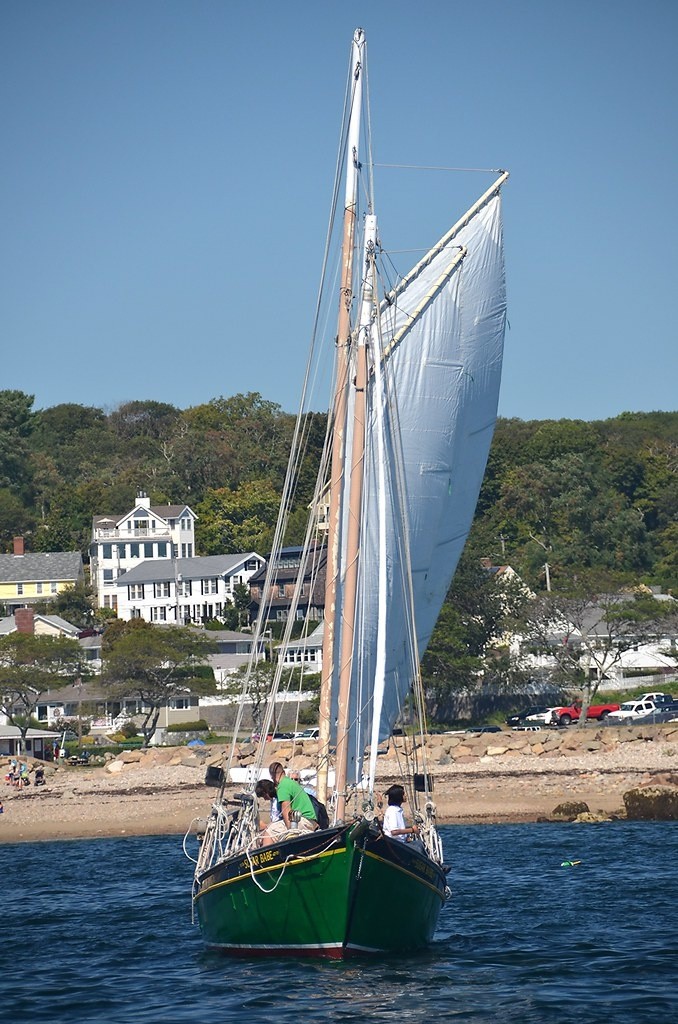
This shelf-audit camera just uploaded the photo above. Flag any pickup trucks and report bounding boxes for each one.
[551,698,620,726]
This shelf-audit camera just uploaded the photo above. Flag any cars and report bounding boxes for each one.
[504,705,544,724]
[525,707,564,725]
[604,701,658,725]
[241,728,319,740]
[636,692,665,701]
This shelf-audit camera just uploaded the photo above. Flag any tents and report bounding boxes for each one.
[187,739,205,746]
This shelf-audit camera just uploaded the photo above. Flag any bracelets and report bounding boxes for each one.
[412,828,414,832]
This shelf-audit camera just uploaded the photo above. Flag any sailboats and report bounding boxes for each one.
[187,23,512,952]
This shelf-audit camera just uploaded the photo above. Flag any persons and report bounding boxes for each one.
[381,784,419,843]
[0,801,3,813]
[255,762,329,847]
[7,759,45,786]
[82,750,90,758]
